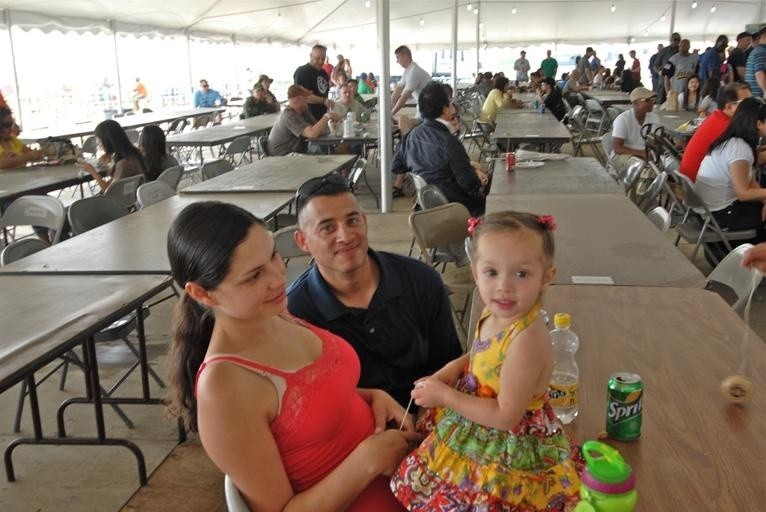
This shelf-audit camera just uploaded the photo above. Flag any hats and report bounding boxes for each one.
[257,74,274,84]
[541,77,555,87]
[630,86,659,104]
[287,84,314,100]
[671,32,681,41]
[248,84,264,96]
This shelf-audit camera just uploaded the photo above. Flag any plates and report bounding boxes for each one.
[516,162,545,168]
[675,129,695,134]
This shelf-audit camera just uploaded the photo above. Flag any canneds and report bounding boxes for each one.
[605,372,644,441]
[342,120,353,138]
[505,152,515,171]
[347,111,356,122]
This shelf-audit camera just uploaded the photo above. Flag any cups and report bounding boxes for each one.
[588,83,615,91]
[328,112,363,139]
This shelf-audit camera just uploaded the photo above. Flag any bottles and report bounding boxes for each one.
[548,312,581,427]
[574,441,639,512]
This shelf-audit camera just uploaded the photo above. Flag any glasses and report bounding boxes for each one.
[294,173,349,220]
[636,96,657,103]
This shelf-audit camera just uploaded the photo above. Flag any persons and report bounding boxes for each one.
[743,242,766,271]
[160,195,420,510]
[278,169,465,434]
[384,209,587,511]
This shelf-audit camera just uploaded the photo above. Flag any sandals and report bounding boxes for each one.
[391,185,405,200]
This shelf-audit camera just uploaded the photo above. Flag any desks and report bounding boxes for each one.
[1,75,765,511]
